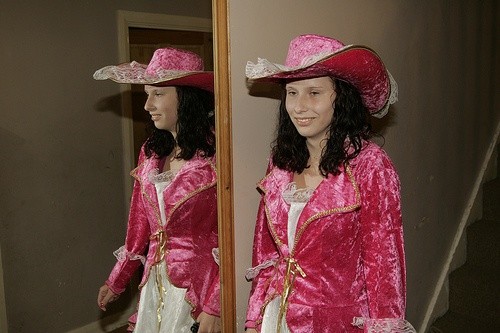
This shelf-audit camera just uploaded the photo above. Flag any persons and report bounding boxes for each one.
[244,34,417,333]
[92,48,222,333]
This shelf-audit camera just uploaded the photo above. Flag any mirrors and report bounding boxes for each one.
[0,0,236,333]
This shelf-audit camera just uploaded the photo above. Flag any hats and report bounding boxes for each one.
[245,34,399,119]
[93,47,214,96]
